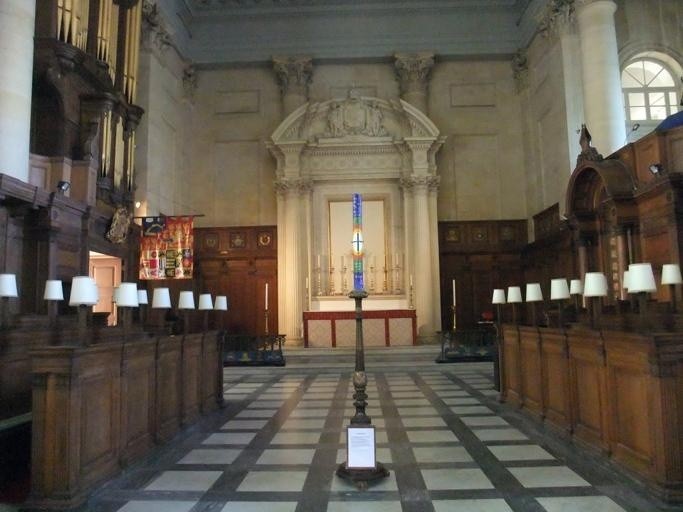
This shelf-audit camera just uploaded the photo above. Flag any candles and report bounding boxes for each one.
[265,283,268,310]
[409,274,412,285]
[340,256,344,267]
[305,278,309,289]
[383,256,385,268]
[371,255,373,268]
[453,279,456,305]
[317,255,320,270]
[330,256,333,268]
[395,252,398,266]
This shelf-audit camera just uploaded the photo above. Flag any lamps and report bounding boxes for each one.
[507,286,522,323]
[661,264,683,312]
[137,290,148,324]
[214,296,228,329]
[152,288,171,337]
[198,294,214,330]
[570,280,582,313]
[0,273,19,330]
[68,276,99,346]
[584,272,608,326]
[112,287,121,326]
[116,283,139,342]
[491,289,506,322]
[178,291,195,334]
[551,278,570,326]
[628,263,657,325]
[526,283,544,326]
[623,270,636,314]
[43,280,64,327]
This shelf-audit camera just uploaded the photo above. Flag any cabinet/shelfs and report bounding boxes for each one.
[493,324,683,504]
[27,328,224,510]
